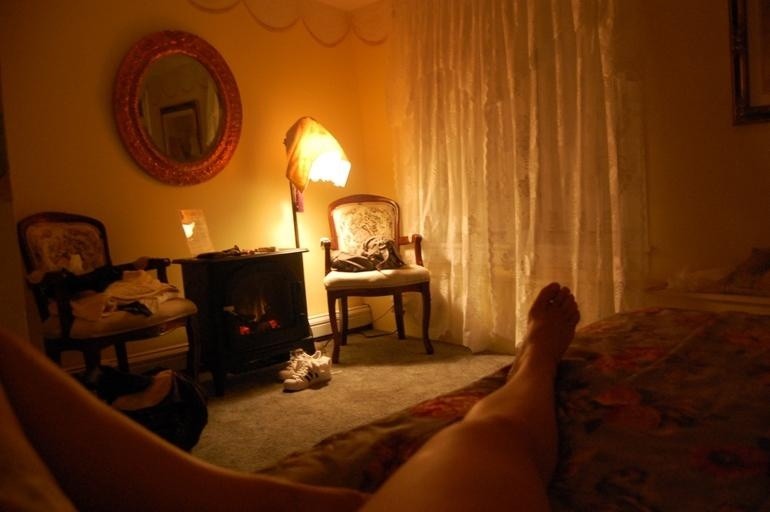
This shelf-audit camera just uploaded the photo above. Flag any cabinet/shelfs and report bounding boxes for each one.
[173,247,316,397]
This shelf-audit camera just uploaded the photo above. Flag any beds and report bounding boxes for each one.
[262,248,768,509]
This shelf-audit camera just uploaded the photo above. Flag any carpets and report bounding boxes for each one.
[319,194,432,366]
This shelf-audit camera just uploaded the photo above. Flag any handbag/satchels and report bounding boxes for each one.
[330,235,405,273]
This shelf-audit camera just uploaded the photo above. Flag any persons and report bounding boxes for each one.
[0,282,581,512]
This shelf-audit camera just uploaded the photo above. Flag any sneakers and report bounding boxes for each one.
[278,348,332,390]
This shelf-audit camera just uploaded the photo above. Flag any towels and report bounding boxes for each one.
[282,116,350,190]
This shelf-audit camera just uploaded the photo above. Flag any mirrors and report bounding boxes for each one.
[111,31,244,187]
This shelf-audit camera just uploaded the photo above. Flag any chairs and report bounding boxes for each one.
[15,209,202,385]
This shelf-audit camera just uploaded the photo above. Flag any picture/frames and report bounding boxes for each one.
[731,4,769,126]
[158,101,203,161]
